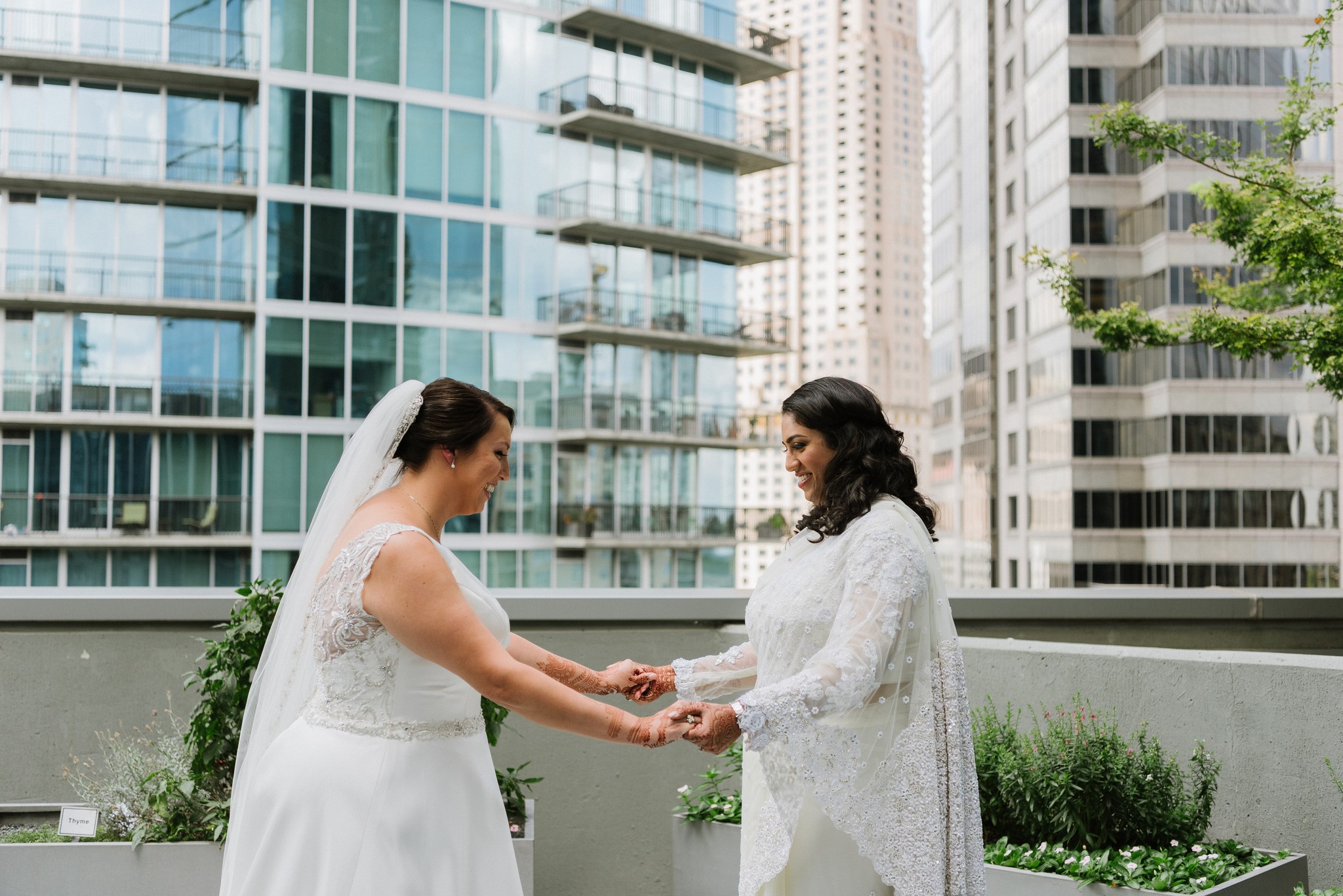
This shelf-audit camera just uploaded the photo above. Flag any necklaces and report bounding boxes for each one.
[397,484,442,545]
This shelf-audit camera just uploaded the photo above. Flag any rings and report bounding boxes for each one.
[625,697,633,700]
[686,714,695,723]
[698,745,704,753]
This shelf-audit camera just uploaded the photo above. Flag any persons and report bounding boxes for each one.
[212,376,702,895]
[606,374,987,896]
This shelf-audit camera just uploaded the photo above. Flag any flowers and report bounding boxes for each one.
[668,689,1296,894]
[0,574,546,853]
